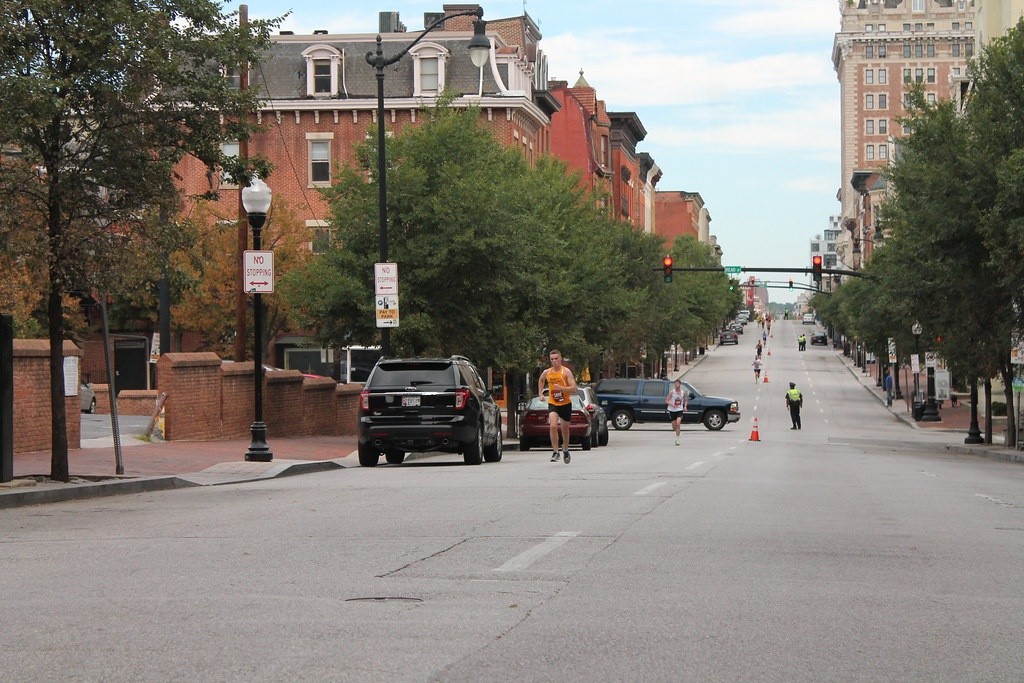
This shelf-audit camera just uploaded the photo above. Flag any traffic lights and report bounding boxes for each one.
[789,281,793,291]
[729,280,734,290]
[663,257,672,283]
[813,256,823,282]
[752,278,754,284]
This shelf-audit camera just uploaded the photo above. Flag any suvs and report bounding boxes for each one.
[358,355,502,467]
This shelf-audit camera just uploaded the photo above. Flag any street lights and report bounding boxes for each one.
[826,257,858,278]
[242,170,273,462]
[366,6,491,361]
[912,320,923,395]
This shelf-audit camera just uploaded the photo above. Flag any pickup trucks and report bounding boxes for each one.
[593,378,741,431]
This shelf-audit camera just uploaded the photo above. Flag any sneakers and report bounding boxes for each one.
[562,449,571,464]
[551,451,560,462]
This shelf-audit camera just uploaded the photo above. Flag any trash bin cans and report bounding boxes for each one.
[915,405,922,422]
[700,347,704,355]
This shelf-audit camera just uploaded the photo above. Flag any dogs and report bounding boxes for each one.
[951,395,957,407]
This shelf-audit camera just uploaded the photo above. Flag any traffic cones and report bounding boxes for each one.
[763,317,776,382]
[748,417,761,441]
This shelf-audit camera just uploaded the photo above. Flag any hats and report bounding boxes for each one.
[789,382,796,386]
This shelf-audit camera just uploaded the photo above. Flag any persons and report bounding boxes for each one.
[763,330,767,347]
[757,313,771,335]
[886,370,892,407]
[786,382,803,430]
[752,356,762,384]
[539,349,576,464]
[665,379,688,445]
[798,334,806,351]
[784,308,788,320]
[755,340,762,356]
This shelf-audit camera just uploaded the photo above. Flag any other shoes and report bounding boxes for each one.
[674,439,680,446]
[791,428,796,430]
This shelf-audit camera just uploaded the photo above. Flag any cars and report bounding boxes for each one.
[720,329,738,345]
[729,310,750,334]
[810,331,828,346]
[80,377,97,414]
[516,395,595,451]
[802,314,815,324]
[542,385,609,448]
